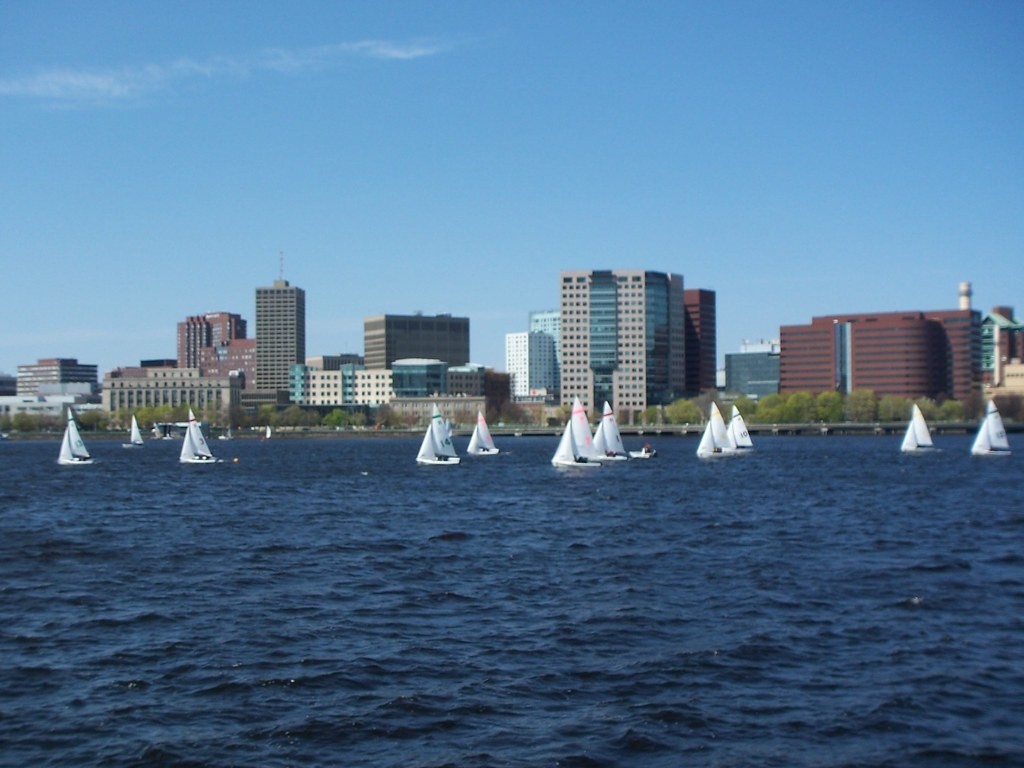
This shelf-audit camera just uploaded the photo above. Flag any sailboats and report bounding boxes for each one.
[724,404,754,452]
[970,399,1012,455]
[466,410,500,455]
[695,401,739,459]
[900,402,938,453]
[590,400,650,459]
[122,414,145,448]
[415,402,461,465]
[55,406,99,464]
[180,409,227,463]
[550,396,608,466]
